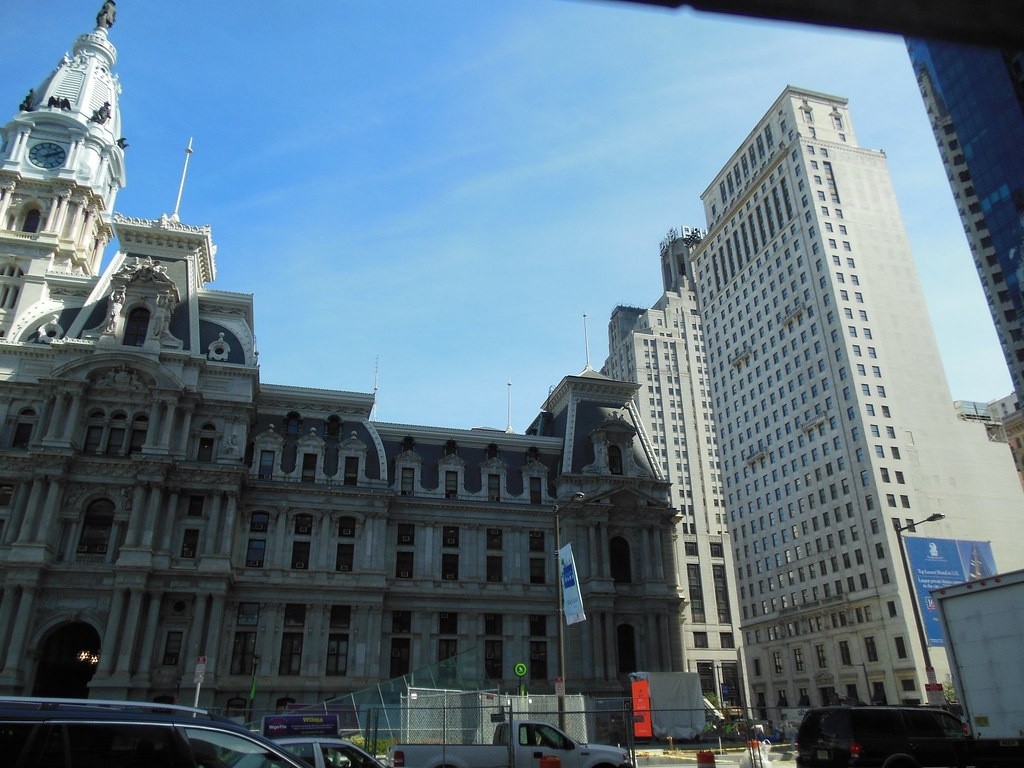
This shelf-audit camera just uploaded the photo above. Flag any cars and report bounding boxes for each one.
[725,706,743,720]
[224,714,389,768]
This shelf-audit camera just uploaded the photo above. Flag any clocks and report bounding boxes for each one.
[24,140,68,171]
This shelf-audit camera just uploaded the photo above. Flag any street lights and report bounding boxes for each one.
[245,656,258,730]
[708,666,724,708]
[896,513,951,706]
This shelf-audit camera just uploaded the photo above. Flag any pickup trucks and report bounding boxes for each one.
[387,720,634,768]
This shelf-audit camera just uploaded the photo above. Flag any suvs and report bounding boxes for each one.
[795,706,971,768]
[0,696,313,768]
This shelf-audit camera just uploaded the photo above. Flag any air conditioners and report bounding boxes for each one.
[614,467,620,473]
[329,647,337,654]
[403,535,410,541]
[296,562,304,569]
[533,531,541,537]
[491,529,499,535]
[77,544,88,552]
[450,494,457,498]
[255,523,263,530]
[97,545,107,553]
[184,550,192,557]
[406,489,413,495]
[299,526,307,533]
[165,655,176,664]
[293,646,301,654]
[340,565,348,571]
[343,529,351,535]
[448,538,455,544]
[250,560,259,567]
[392,570,539,660]
